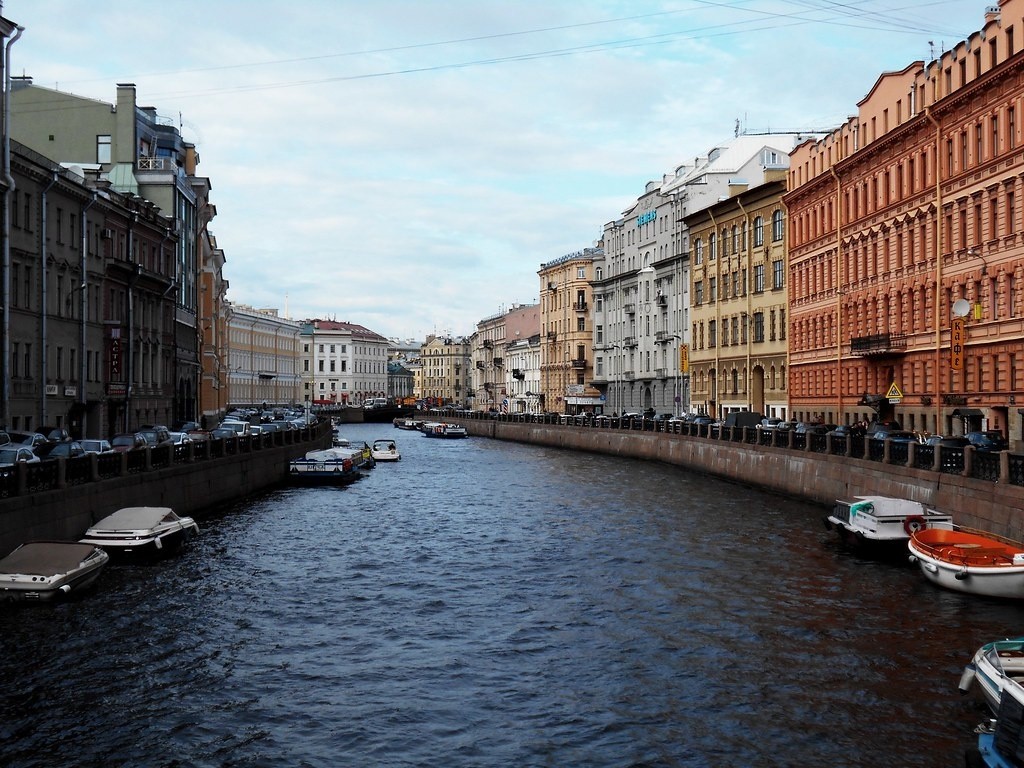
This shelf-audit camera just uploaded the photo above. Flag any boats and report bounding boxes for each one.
[907,521,1024,599]
[826,494,953,542]
[420,422,468,439]
[954,637,1024,768]
[288,416,371,477]
[393,417,431,430]
[371,439,401,461]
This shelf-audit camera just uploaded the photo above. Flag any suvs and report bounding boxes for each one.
[924,435,973,467]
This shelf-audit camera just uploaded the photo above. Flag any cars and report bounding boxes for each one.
[138,430,174,448]
[33,441,87,462]
[34,426,72,447]
[866,420,902,436]
[170,432,193,451]
[761,417,839,446]
[965,432,1009,451]
[0,447,40,474]
[212,408,317,439]
[7,429,49,452]
[0,430,12,448]
[824,425,859,446]
[188,431,214,443]
[182,423,203,434]
[110,432,147,453]
[431,404,726,429]
[76,440,114,456]
[872,430,921,454]
